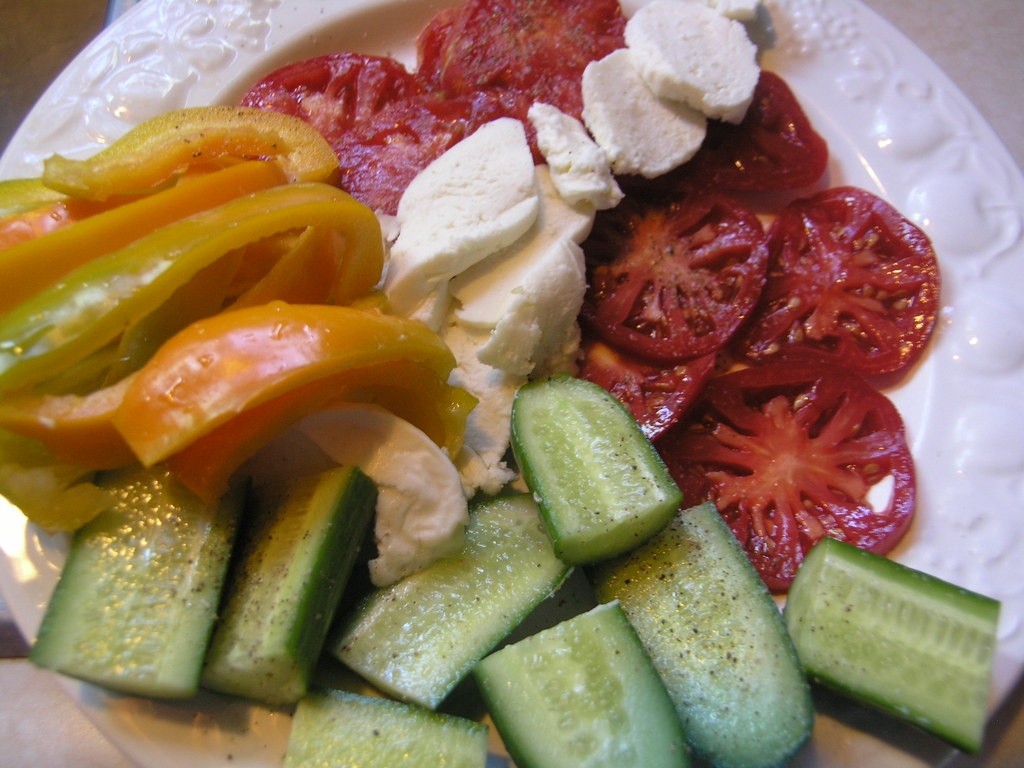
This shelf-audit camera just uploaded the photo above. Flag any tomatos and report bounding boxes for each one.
[232,0,941,593]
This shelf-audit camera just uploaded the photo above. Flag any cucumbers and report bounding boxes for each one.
[30,374,1001,768]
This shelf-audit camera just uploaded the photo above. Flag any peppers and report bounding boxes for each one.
[1,107,481,533]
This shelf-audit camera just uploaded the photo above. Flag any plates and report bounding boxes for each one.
[1,0,1024,768]
